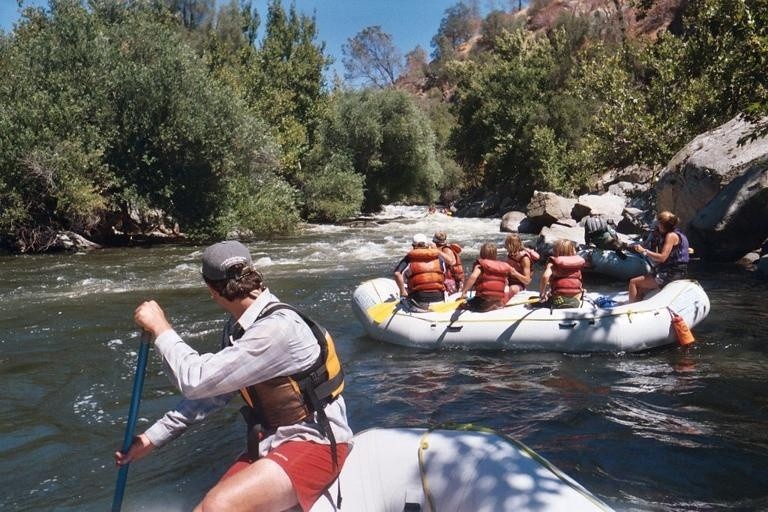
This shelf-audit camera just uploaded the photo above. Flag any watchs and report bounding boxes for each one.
[642,249,648,256]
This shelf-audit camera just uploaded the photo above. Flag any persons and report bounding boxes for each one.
[432,232,464,294]
[428,205,452,214]
[585,213,634,261]
[461,244,515,310]
[394,234,446,304]
[529,238,585,308]
[113,239,354,512]
[628,210,689,304]
[504,234,540,295]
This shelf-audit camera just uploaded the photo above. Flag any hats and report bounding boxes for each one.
[202,241,257,280]
[412,234,426,246]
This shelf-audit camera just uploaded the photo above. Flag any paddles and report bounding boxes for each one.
[368,297,406,323]
[427,298,540,311]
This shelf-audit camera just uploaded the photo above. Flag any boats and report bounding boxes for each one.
[439,210,453,216]
[571,241,658,283]
[308,418,619,512]
[352,276,712,353]
